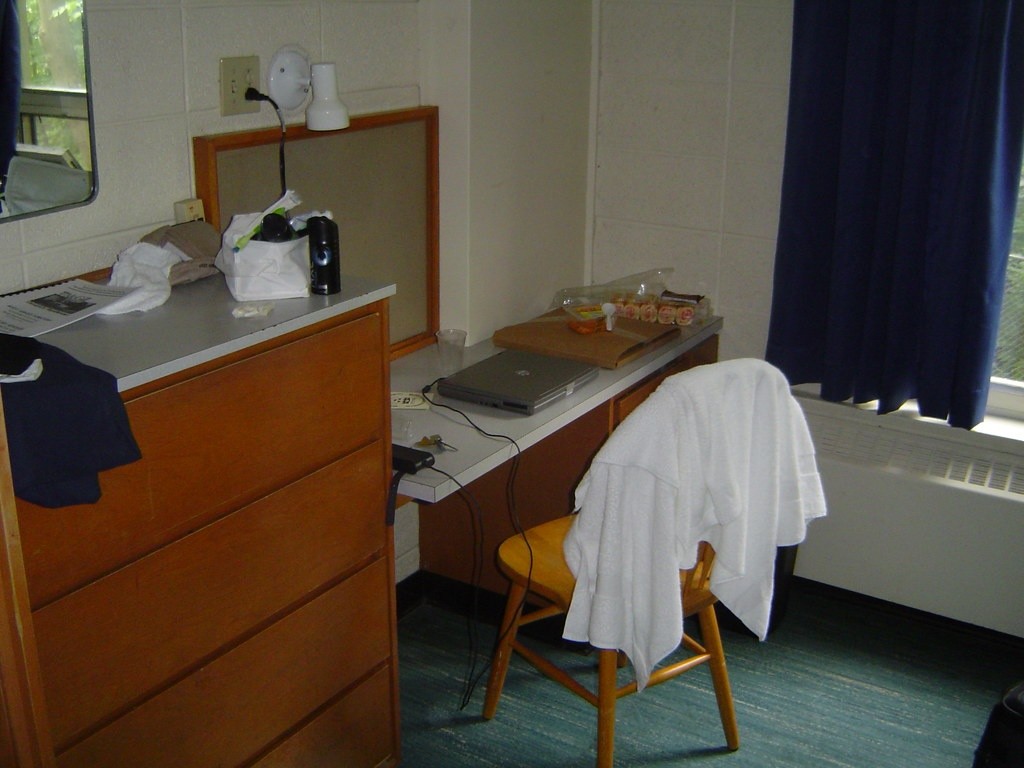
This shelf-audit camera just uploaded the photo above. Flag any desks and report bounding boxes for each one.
[390,302,723,601]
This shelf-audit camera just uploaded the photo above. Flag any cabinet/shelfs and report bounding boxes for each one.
[0,257,398,768]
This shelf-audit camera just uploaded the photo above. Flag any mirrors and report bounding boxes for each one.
[0,0,99,225]
[192,105,441,362]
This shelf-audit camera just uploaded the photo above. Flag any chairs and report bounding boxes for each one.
[480,513,741,768]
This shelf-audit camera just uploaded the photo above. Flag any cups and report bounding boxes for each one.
[435,328,468,377]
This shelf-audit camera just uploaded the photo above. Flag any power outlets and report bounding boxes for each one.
[220,56,263,117]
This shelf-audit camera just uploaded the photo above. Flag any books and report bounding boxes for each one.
[0,278,142,337]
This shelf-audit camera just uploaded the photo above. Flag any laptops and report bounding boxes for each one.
[437,348,601,415]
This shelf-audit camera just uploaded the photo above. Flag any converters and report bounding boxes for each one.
[391,443,435,477]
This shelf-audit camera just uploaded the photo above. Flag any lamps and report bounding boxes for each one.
[267,44,350,131]
